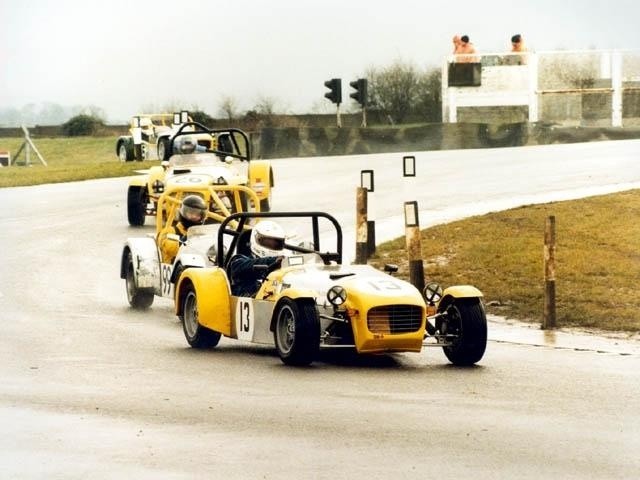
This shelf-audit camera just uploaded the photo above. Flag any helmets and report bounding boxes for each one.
[249,219,287,258]
[180,194,208,227]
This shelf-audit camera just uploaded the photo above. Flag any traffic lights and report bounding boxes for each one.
[350,78,368,105]
[324,77,342,104]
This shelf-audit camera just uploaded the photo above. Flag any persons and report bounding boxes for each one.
[453,36,460,47]
[452,35,474,53]
[226,220,285,297]
[140,119,154,142]
[158,195,207,264]
[511,34,523,52]
